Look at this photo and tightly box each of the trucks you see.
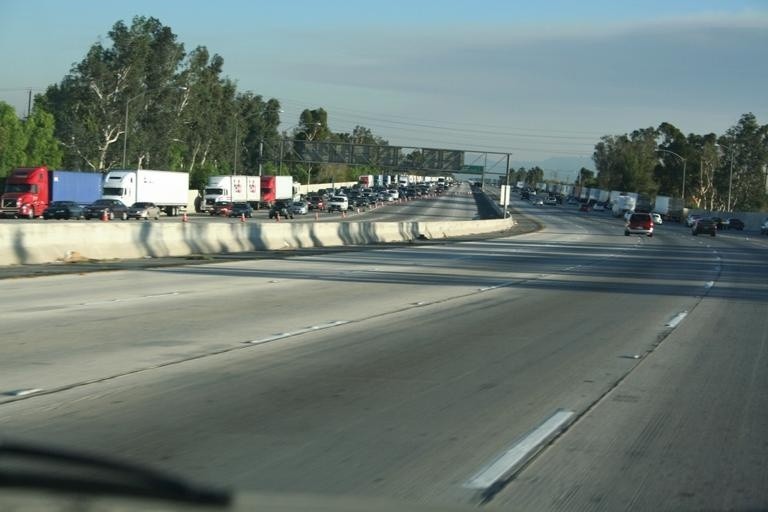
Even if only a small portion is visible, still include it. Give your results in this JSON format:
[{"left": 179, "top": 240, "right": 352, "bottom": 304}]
[
  {"left": 199, "top": 175, "right": 292, "bottom": 213},
  {"left": 359, "top": 175, "right": 453, "bottom": 188},
  {"left": 102, "top": 169, "right": 189, "bottom": 216},
  {"left": 0, "top": 166, "right": 104, "bottom": 218}
]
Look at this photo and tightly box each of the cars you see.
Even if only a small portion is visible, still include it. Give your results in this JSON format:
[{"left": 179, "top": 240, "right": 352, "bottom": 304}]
[
  {"left": 43, "top": 199, "right": 161, "bottom": 220},
  {"left": 269, "top": 182, "right": 464, "bottom": 219},
  {"left": 510, "top": 186, "right": 768, "bottom": 237},
  {"left": 210, "top": 202, "right": 253, "bottom": 218}
]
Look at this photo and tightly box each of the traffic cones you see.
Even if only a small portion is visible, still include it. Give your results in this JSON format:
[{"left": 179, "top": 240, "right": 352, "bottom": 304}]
[
  {"left": 183, "top": 212, "right": 188, "bottom": 222},
  {"left": 276, "top": 213, "right": 281, "bottom": 222},
  {"left": 241, "top": 213, "right": 245, "bottom": 222},
  {"left": 314, "top": 212, "right": 319, "bottom": 220},
  {"left": 101, "top": 211, "right": 108, "bottom": 222}
]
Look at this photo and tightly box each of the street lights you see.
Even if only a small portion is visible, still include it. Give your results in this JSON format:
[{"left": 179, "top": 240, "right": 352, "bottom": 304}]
[
  {"left": 713, "top": 143, "right": 734, "bottom": 212},
  {"left": 654, "top": 148, "right": 686, "bottom": 199},
  {"left": 279, "top": 122, "right": 322, "bottom": 175},
  {"left": 233, "top": 109, "right": 285, "bottom": 175},
  {"left": 121, "top": 87, "right": 188, "bottom": 169}
]
